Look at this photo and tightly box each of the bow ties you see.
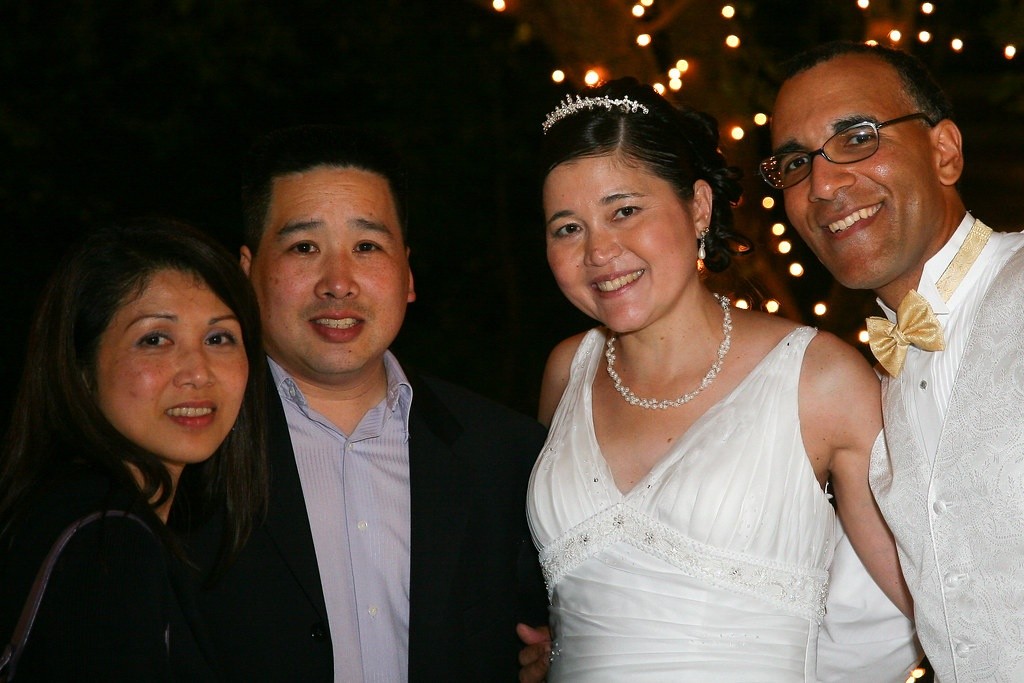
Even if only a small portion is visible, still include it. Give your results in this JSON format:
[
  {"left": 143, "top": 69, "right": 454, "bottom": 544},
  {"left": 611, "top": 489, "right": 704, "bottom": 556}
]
[{"left": 866, "top": 218, "right": 993, "bottom": 378}]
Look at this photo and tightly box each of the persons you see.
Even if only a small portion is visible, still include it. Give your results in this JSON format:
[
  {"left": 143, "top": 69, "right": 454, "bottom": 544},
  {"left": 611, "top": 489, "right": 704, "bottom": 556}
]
[
  {"left": 0, "top": 217, "right": 272, "bottom": 683},
  {"left": 166, "top": 124, "right": 550, "bottom": 683},
  {"left": 523, "top": 76, "right": 914, "bottom": 682},
  {"left": 516, "top": 44, "right": 1024, "bottom": 682}
]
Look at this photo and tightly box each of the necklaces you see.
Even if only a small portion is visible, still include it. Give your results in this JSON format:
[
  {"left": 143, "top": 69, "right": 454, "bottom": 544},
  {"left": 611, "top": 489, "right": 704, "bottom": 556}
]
[{"left": 606, "top": 294, "right": 730, "bottom": 410}]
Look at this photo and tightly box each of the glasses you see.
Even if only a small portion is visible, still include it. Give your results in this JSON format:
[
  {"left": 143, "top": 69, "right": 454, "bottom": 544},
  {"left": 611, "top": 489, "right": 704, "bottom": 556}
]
[{"left": 758, "top": 112, "right": 934, "bottom": 190}]
